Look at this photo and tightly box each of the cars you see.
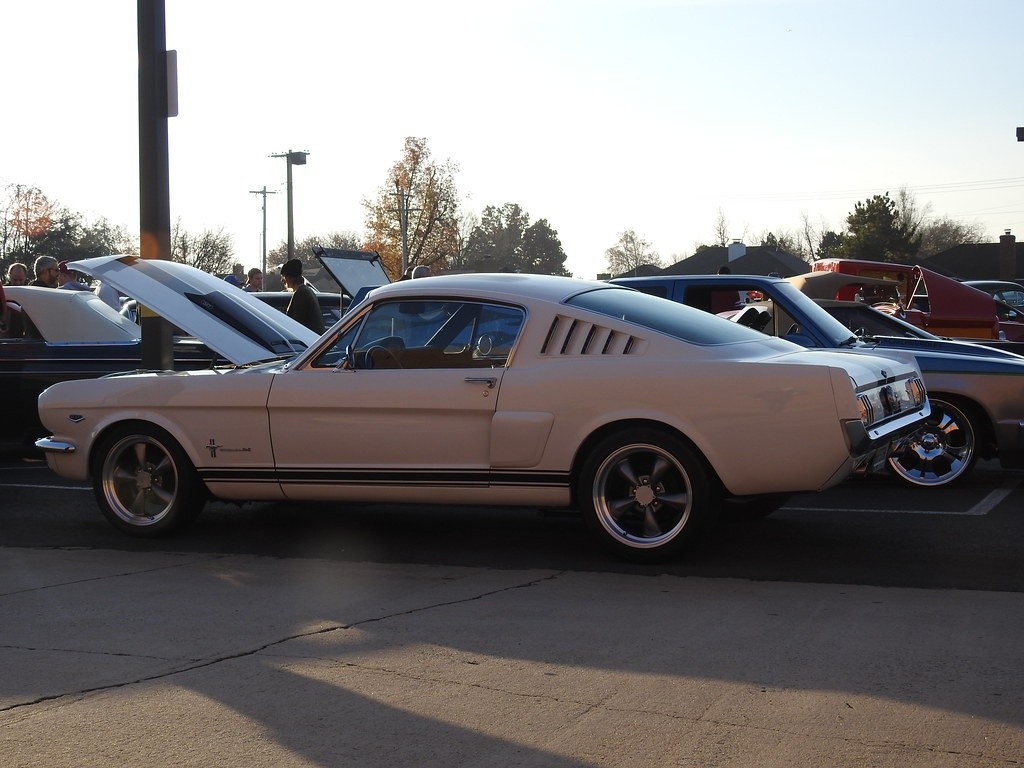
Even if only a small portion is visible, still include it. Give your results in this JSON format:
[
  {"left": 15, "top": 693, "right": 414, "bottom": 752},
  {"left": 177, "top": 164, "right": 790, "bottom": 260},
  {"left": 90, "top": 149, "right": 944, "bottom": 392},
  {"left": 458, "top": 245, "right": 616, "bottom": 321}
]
[
  {"left": 714, "top": 257, "right": 1023, "bottom": 356},
  {"left": 34, "top": 254, "right": 932, "bottom": 540},
  {"left": 0, "top": 286, "right": 231, "bottom": 432},
  {"left": 608, "top": 275, "right": 1023, "bottom": 488}
]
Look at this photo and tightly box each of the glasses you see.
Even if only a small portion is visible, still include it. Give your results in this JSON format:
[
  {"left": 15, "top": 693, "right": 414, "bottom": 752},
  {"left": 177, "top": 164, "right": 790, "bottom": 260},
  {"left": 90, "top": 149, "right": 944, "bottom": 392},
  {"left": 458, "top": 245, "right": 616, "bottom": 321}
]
[{"left": 50, "top": 268, "right": 60, "bottom": 272}]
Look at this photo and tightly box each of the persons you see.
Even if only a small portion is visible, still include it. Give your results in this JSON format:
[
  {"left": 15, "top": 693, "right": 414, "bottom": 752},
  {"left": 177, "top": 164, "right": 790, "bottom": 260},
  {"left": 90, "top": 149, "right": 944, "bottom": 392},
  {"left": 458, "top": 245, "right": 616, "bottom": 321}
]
[
  {"left": 411, "top": 266, "right": 430, "bottom": 279},
  {"left": 20, "top": 256, "right": 59, "bottom": 338},
  {"left": 222, "top": 275, "right": 244, "bottom": 289},
  {"left": 56, "top": 259, "right": 95, "bottom": 292},
  {"left": 241, "top": 268, "right": 262, "bottom": 292},
  {"left": 712, "top": 266, "right": 740, "bottom": 314},
  {"left": 0, "top": 263, "right": 30, "bottom": 337},
  {"left": 395, "top": 266, "right": 414, "bottom": 282},
  {"left": 278, "top": 259, "right": 323, "bottom": 333}
]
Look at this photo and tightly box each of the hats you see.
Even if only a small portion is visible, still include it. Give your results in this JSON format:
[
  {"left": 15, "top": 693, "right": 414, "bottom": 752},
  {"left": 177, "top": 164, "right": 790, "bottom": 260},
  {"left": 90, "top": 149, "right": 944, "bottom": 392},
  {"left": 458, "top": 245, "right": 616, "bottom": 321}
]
[{"left": 280, "top": 258, "right": 303, "bottom": 275}]
